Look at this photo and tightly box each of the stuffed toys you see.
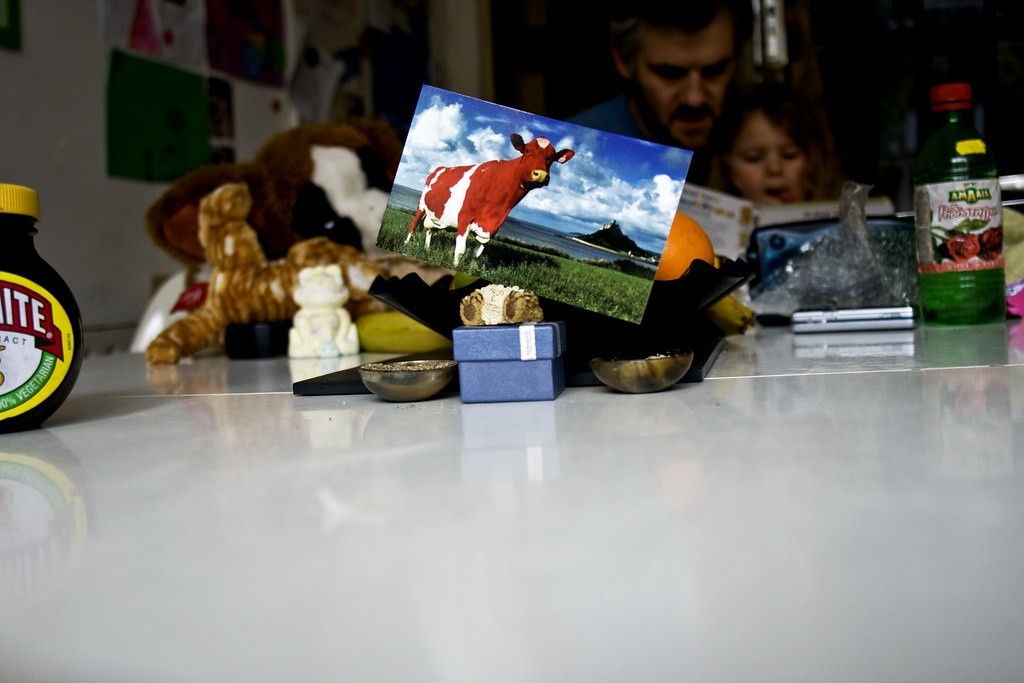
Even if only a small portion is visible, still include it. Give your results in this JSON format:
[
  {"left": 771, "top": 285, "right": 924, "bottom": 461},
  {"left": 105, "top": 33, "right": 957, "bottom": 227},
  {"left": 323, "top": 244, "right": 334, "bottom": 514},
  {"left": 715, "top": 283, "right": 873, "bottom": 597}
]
[{"left": 144, "top": 118, "right": 404, "bottom": 369}]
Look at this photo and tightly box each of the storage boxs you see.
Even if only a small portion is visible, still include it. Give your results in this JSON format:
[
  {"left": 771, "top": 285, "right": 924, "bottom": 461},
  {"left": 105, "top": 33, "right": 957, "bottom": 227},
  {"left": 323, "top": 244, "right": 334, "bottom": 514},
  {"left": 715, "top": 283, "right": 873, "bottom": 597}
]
[{"left": 453, "top": 320, "right": 563, "bottom": 404}]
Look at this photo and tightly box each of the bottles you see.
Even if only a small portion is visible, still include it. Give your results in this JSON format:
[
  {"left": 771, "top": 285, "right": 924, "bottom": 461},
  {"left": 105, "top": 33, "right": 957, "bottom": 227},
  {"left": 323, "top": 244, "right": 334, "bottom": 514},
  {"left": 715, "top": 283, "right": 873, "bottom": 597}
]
[
  {"left": 912, "top": 82, "right": 1006, "bottom": 325},
  {"left": 0, "top": 183, "right": 84, "bottom": 434}
]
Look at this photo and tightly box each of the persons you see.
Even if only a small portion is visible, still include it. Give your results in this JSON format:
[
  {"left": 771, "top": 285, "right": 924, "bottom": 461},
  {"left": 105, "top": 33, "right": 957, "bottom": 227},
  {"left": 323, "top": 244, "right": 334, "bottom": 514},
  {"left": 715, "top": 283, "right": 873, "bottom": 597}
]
[{"left": 567, "top": 1, "right": 844, "bottom": 207}]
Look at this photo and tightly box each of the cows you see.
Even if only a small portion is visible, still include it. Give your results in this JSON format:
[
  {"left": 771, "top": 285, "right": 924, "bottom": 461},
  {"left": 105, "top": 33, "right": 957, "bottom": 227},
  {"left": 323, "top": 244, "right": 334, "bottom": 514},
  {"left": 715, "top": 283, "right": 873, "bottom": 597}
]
[{"left": 403, "top": 133, "right": 575, "bottom": 267}]
